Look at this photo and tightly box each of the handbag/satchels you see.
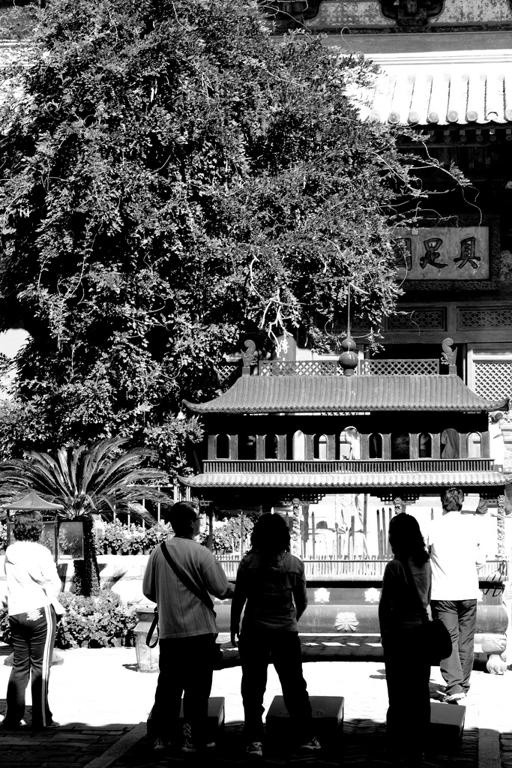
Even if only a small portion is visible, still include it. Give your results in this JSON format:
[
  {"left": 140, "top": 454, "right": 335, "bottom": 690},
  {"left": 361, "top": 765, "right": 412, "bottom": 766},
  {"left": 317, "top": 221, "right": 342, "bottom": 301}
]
[{"left": 420, "top": 617, "right": 452, "bottom": 665}]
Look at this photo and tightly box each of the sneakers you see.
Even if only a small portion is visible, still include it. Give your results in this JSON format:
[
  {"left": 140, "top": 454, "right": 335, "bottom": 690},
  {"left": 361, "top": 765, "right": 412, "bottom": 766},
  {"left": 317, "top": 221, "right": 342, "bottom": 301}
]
[
  {"left": 246, "top": 742, "right": 263, "bottom": 756},
  {"left": 442, "top": 684, "right": 465, "bottom": 702},
  {"left": 303, "top": 736, "right": 322, "bottom": 749}
]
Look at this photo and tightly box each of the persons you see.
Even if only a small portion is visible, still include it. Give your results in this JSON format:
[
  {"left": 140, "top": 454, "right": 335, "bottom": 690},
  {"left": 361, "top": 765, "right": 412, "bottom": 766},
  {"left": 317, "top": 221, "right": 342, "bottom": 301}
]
[
  {"left": 424, "top": 487, "right": 485, "bottom": 704},
  {"left": 0, "top": 511, "right": 62, "bottom": 731},
  {"left": 141, "top": 503, "right": 228, "bottom": 750},
  {"left": 375, "top": 513, "right": 433, "bottom": 756},
  {"left": 230, "top": 512, "right": 324, "bottom": 756}
]
